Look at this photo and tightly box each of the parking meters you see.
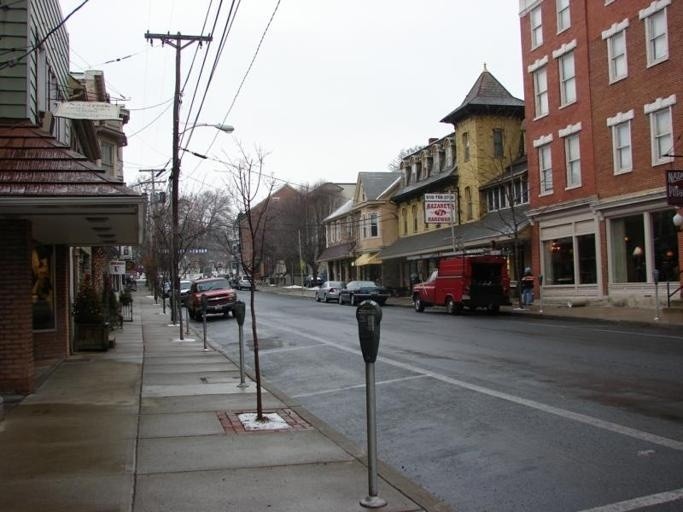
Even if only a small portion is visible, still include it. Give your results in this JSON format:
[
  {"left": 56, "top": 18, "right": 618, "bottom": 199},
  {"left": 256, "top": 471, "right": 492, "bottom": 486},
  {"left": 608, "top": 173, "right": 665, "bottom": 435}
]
[
  {"left": 538, "top": 275, "right": 544, "bottom": 313},
  {"left": 356, "top": 299, "right": 387, "bottom": 508},
  {"left": 232, "top": 300, "right": 246, "bottom": 386},
  {"left": 652, "top": 269, "right": 659, "bottom": 317},
  {"left": 201, "top": 294, "right": 208, "bottom": 349}
]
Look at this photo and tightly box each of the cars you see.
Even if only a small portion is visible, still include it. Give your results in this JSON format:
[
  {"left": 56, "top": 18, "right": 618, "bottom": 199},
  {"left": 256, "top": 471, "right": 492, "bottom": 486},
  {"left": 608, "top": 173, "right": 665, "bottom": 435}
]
[
  {"left": 160, "top": 277, "right": 237, "bottom": 320},
  {"left": 230, "top": 275, "right": 257, "bottom": 291},
  {"left": 303, "top": 275, "right": 325, "bottom": 288},
  {"left": 315, "top": 280, "right": 391, "bottom": 306}
]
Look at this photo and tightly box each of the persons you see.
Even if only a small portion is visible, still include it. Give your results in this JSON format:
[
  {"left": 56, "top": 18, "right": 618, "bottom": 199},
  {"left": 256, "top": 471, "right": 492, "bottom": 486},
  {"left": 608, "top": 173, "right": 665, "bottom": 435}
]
[{"left": 521, "top": 267, "right": 534, "bottom": 305}]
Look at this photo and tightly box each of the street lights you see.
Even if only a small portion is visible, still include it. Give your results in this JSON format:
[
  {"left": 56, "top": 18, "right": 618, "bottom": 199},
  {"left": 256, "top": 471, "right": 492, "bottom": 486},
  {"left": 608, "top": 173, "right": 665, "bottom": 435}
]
[{"left": 178, "top": 124, "right": 234, "bottom": 139}]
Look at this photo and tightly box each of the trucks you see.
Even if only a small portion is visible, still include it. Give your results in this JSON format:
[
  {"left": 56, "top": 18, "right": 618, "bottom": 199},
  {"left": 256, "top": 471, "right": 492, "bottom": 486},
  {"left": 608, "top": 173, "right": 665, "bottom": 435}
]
[{"left": 411, "top": 255, "right": 512, "bottom": 315}]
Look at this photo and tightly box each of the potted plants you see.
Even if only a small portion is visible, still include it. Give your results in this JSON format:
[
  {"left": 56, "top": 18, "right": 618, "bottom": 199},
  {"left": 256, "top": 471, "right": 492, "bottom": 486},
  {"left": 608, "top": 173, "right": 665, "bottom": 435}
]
[{"left": 72, "top": 287, "right": 109, "bottom": 352}]
